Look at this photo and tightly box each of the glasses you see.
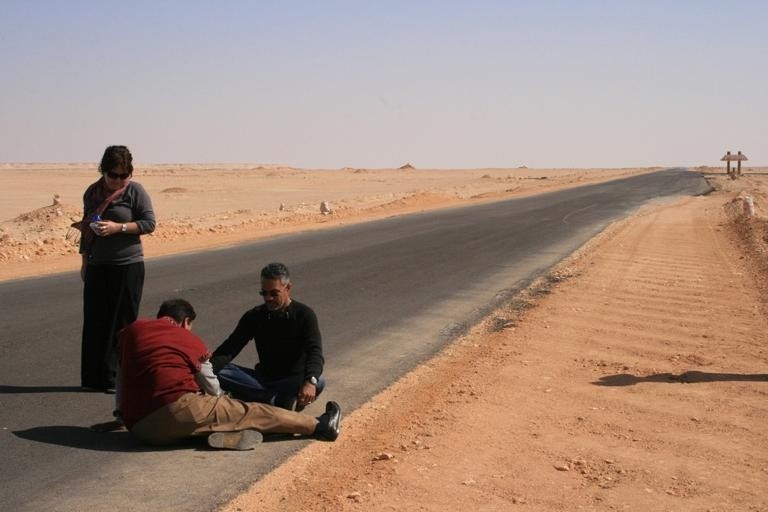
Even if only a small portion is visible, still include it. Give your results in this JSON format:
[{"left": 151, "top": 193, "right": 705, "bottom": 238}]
[
  {"left": 105, "top": 169, "right": 132, "bottom": 181},
  {"left": 258, "top": 283, "right": 289, "bottom": 298}
]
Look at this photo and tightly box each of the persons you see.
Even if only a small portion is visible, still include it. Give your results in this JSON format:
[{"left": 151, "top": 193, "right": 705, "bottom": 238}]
[
  {"left": 209, "top": 261, "right": 326, "bottom": 412},
  {"left": 90, "top": 299, "right": 342, "bottom": 451},
  {"left": 76, "top": 144, "right": 157, "bottom": 394}
]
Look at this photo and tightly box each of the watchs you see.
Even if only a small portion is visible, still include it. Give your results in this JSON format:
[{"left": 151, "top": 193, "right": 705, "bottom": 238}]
[{"left": 310, "top": 376, "right": 318, "bottom": 384}]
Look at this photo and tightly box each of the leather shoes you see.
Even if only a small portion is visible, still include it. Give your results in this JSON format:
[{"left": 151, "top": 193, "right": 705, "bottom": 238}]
[
  {"left": 206, "top": 429, "right": 264, "bottom": 452},
  {"left": 324, "top": 401, "right": 342, "bottom": 442},
  {"left": 275, "top": 392, "right": 298, "bottom": 413},
  {"left": 106, "top": 388, "right": 116, "bottom": 394}
]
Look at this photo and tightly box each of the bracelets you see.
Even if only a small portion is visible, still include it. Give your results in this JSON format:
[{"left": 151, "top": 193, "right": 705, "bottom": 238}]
[{"left": 122, "top": 224, "right": 128, "bottom": 232}]
